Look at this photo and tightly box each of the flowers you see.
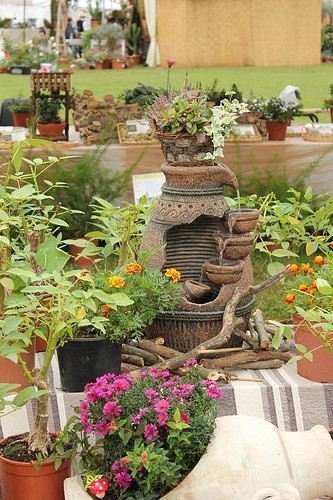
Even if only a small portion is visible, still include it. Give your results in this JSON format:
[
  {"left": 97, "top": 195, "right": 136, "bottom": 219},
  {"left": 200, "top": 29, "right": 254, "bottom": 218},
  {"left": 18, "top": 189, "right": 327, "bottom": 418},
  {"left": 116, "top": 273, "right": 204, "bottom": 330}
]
[
  {"left": 0, "top": 139, "right": 333, "bottom": 500},
  {"left": 248, "top": 94, "right": 300, "bottom": 125},
  {"left": 144, "top": 59, "right": 250, "bottom": 160}
]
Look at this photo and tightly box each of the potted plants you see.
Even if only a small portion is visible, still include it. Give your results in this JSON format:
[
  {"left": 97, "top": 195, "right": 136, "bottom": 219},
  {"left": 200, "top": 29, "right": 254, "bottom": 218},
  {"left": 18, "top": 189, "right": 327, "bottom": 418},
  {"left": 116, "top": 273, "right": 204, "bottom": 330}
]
[
  {"left": 36, "top": 98, "right": 68, "bottom": 136},
  {"left": 321, "top": 32, "right": 333, "bottom": 63},
  {"left": 1, "top": 1, "right": 150, "bottom": 74},
  {"left": 8, "top": 90, "right": 36, "bottom": 128}
]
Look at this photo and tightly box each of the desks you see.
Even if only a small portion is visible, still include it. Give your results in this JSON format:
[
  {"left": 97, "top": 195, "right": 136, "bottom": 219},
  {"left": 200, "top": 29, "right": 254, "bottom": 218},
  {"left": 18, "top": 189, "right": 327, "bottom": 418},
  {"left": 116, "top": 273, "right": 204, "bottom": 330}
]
[
  {"left": 0, "top": 125, "right": 333, "bottom": 206},
  {"left": 0, "top": 324, "right": 333, "bottom": 441}
]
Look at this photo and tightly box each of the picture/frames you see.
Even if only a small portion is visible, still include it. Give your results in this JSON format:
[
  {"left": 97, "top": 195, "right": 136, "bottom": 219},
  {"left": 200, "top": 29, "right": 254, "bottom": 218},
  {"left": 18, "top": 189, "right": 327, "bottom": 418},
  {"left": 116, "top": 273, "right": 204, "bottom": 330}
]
[{"left": 268, "top": 121, "right": 287, "bottom": 141}]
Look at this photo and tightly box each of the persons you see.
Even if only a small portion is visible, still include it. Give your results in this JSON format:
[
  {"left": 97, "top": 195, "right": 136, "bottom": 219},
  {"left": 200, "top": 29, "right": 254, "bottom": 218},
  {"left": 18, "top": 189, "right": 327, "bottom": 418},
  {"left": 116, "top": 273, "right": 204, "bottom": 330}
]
[
  {"left": 65, "top": 17, "right": 77, "bottom": 59},
  {"left": 39, "top": 30, "right": 46, "bottom": 39},
  {"left": 77, "top": 13, "right": 86, "bottom": 58}
]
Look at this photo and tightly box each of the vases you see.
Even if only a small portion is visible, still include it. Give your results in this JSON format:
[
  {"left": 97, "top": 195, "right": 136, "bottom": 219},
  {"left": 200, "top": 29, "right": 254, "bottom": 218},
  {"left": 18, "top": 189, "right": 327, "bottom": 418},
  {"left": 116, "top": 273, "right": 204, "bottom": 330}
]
[
  {"left": 0, "top": 432, "right": 73, "bottom": 500},
  {"left": 0, "top": 335, "right": 36, "bottom": 394},
  {"left": 22, "top": 293, "right": 58, "bottom": 352},
  {"left": 291, "top": 313, "right": 333, "bottom": 383},
  {"left": 57, "top": 336, "right": 123, "bottom": 393},
  {"left": 68, "top": 237, "right": 99, "bottom": 267},
  {"left": 155, "top": 131, "right": 217, "bottom": 167}
]
